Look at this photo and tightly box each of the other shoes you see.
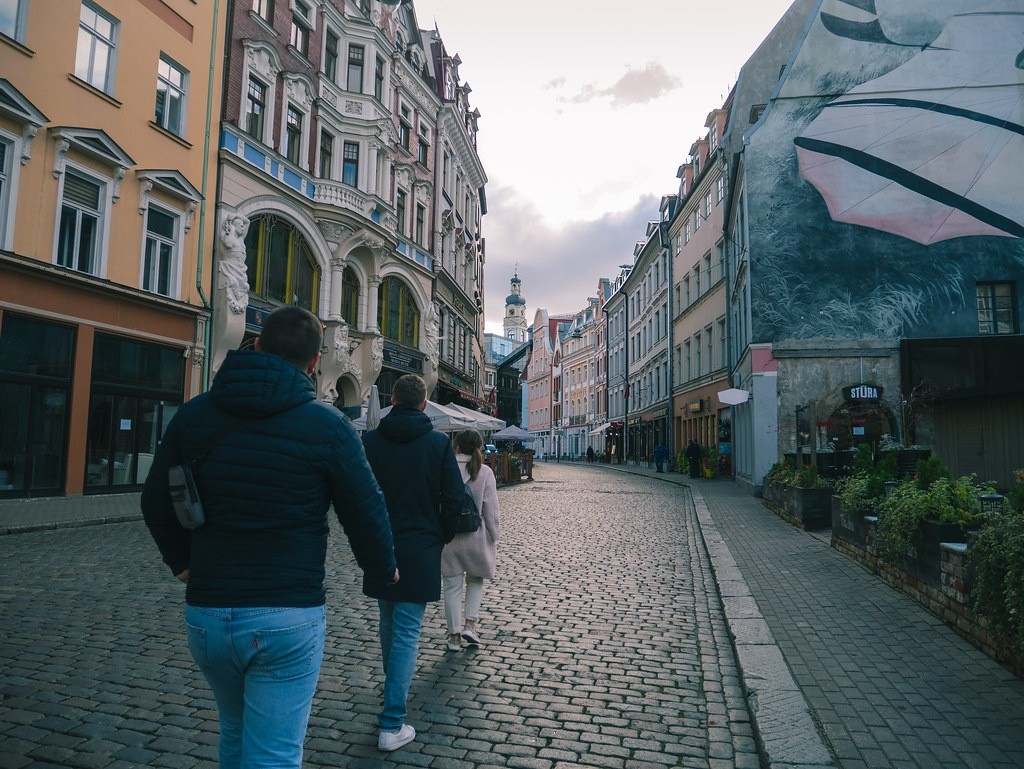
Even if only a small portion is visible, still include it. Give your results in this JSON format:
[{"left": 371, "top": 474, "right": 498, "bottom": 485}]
[
  {"left": 462, "top": 630, "right": 480, "bottom": 644},
  {"left": 446, "top": 639, "right": 460, "bottom": 651}
]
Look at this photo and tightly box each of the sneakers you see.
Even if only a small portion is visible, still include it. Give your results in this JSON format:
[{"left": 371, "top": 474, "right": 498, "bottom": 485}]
[{"left": 378, "top": 722, "right": 416, "bottom": 751}]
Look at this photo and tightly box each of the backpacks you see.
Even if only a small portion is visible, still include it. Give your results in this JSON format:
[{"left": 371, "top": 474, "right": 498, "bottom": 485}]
[{"left": 455, "top": 484, "right": 482, "bottom": 533}]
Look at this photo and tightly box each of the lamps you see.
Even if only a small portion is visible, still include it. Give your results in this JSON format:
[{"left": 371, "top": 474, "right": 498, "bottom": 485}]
[{"left": 347, "top": 341, "right": 361, "bottom": 356}]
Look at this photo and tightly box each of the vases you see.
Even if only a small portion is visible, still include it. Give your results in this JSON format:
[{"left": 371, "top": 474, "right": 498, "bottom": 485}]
[
  {"left": 888, "top": 450, "right": 932, "bottom": 480},
  {"left": 885, "top": 481, "right": 901, "bottom": 498},
  {"left": 921, "top": 516, "right": 981, "bottom": 554}
]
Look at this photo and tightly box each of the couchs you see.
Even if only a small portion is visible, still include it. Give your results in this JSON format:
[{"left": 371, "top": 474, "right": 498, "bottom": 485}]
[{"left": 102, "top": 453, "right": 154, "bottom": 485}]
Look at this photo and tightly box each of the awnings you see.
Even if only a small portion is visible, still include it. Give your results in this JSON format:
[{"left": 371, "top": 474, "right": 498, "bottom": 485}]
[{"left": 589, "top": 422, "right": 612, "bottom": 436}]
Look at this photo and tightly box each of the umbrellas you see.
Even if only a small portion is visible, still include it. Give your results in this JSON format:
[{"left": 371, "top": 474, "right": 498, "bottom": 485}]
[
  {"left": 351, "top": 399, "right": 478, "bottom": 432},
  {"left": 491, "top": 425, "right": 535, "bottom": 455},
  {"left": 444, "top": 402, "right": 506, "bottom": 449}
]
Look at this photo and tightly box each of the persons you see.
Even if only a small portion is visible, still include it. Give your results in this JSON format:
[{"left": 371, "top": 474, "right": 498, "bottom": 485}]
[
  {"left": 692, "top": 439, "right": 702, "bottom": 478},
  {"left": 687, "top": 439, "right": 696, "bottom": 478},
  {"left": 358, "top": 373, "right": 465, "bottom": 752},
  {"left": 653, "top": 442, "right": 665, "bottom": 474},
  {"left": 586, "top": 445, "right": 594, "bottom": 464},
  {"left": 140, "top": 307, "right": 400, "bottom": 769},
  {"left": 441, "top": 429, "right": 500, "bottom": 651}
]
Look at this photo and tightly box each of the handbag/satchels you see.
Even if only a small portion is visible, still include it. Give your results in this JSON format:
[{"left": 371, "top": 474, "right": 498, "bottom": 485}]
[{"left": 167, "top": 463, "right": 205, "bottom": 530}]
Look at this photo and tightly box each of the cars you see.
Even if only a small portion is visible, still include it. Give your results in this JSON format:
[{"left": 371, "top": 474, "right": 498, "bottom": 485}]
[{"left": 485, "top": 445, "right": 499, "bottom": 460}]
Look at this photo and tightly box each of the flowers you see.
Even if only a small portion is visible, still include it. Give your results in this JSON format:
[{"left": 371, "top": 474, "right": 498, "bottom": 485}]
[
  {"left": 872, "top": 472, "right": 1001, "bottom": 557},
  {"left": 878, "top": 433, "right": 934, "bottom": 451}
]
